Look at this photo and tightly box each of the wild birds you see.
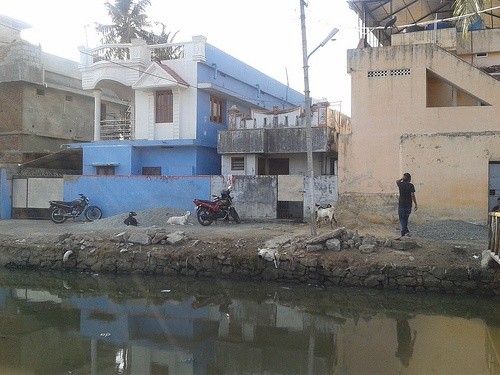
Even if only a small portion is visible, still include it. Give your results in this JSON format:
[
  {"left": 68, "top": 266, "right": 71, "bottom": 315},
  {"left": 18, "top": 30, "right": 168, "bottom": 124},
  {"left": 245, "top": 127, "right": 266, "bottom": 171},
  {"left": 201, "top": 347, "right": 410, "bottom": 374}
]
[{"left": 384, "top": 16, "right": 397, "bottom": 30}]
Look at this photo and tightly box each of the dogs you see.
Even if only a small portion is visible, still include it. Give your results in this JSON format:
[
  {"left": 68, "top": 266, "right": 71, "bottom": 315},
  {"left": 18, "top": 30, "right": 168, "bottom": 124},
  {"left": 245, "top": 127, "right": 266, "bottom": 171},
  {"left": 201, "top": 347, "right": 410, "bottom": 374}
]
[
  {"left": 124, "top": 211, "right": 139, "bottom": 226},
  {"left": 315, "top": 203, "right": 338, "bottom": 229},
  {"left": 167, "top": 211, "right": 193, "bottom": 226}
]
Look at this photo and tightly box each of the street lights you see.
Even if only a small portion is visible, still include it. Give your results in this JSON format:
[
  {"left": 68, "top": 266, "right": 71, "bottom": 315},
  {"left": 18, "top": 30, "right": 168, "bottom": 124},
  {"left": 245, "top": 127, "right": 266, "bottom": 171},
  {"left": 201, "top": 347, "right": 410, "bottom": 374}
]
[{"left": 299, "top": 0, "right": 340, "bottom": 241}]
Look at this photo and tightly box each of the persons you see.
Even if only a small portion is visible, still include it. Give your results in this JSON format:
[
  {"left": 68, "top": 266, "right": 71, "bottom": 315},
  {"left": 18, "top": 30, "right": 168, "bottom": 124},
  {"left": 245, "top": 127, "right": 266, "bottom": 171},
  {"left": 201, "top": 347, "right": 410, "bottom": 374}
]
[
  {"left": 491, "top": 198, "right": 500, "bottom": 211},
  {"left": 396, "top": 172, "right": 418, "bottom": 240}
]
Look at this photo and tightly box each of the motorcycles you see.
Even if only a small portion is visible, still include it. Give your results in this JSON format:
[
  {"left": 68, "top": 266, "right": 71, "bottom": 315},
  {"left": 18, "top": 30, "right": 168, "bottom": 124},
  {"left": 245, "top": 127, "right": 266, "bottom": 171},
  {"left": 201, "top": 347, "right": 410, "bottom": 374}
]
[
  {"left": 193, "top": 185, "right": 242, "bottom": 226},
  {"left": 48, "top": 193, "right": 104, "bottom": 223}
]
